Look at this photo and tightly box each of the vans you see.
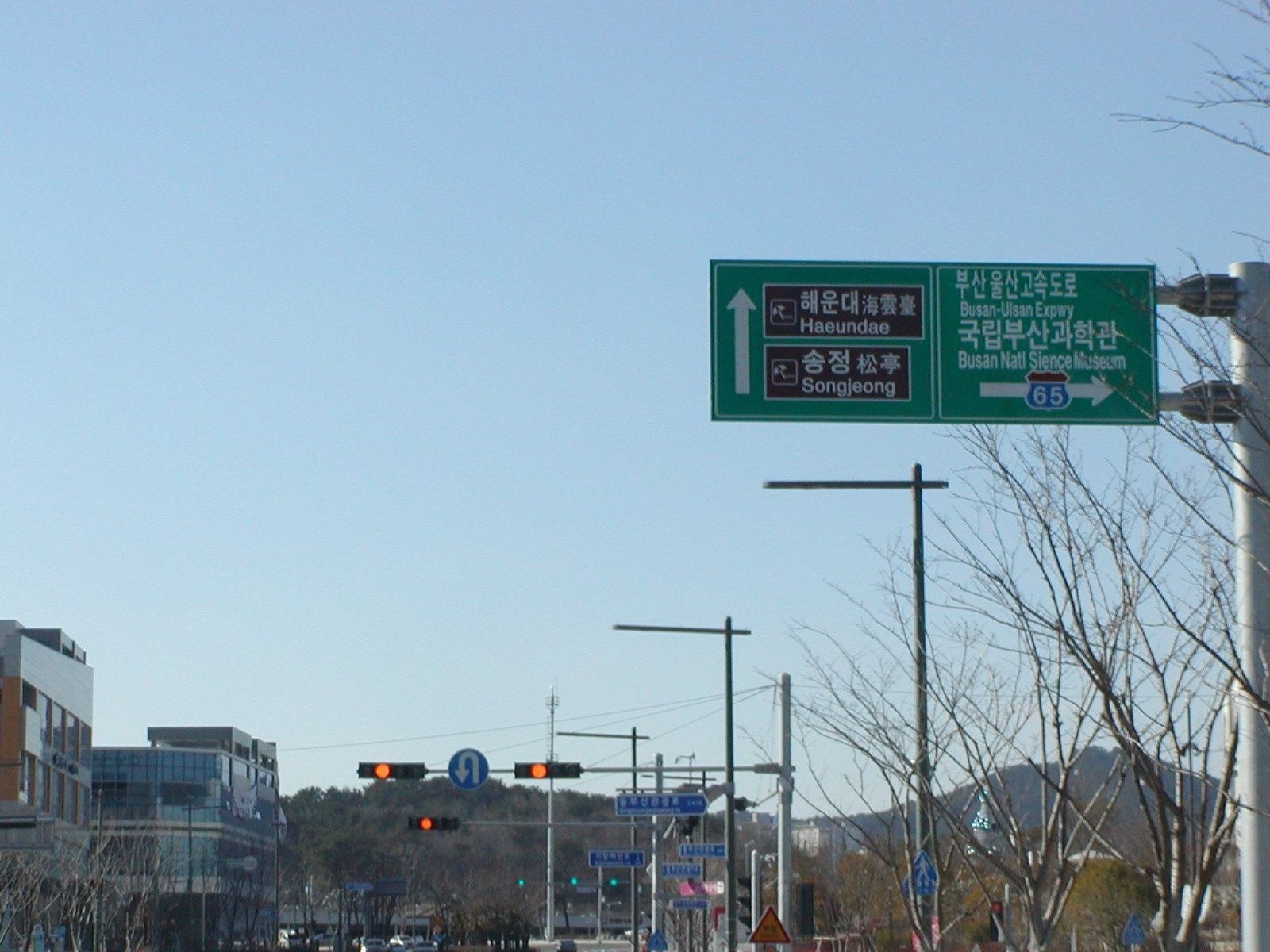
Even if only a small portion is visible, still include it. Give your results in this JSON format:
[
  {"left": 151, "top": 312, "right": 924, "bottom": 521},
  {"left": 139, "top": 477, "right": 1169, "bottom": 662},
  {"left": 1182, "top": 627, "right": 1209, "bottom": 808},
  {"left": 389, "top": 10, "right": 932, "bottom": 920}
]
[{"left": 277, "top": 927, "right": 310, "bottom": 952}]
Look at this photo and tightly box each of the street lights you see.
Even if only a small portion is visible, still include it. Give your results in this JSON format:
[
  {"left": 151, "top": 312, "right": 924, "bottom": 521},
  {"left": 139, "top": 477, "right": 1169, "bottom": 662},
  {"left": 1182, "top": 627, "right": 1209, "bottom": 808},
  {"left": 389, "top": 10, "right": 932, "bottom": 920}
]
[
  {"left": 611, "top": 616, "right": 754, "bottom": 952},
  {"left": 555, "top": 726, "right": 651, "bottom": 952},
  {"left": 760, "top": 462, "right": 949, "bottom": 952},
  {"left": 269, "top": 818, "right": 325, "bottom": 952},
  {"left": 182, "top": 801, "right": 234, "bottom": 952}
]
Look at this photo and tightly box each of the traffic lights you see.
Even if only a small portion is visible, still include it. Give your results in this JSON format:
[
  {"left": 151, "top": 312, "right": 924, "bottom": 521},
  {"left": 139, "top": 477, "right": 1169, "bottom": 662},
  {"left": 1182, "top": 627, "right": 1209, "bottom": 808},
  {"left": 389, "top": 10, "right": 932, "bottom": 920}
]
[
  {"left": 408, "top": 816, "right": 459, "bottom": 831},
  {"left": 357, "top": 761, "right": 425, "bottom": 780},
  {"left": 514, "top": 762, "right": 581, "bottom": 779}
]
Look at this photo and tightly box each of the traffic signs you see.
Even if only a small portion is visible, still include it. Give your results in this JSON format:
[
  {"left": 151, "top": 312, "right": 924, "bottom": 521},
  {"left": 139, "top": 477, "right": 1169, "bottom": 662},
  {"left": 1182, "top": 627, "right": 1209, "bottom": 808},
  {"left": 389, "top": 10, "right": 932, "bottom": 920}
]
[{"left": 707, "top": 257, "right": 1161, "bottom": 428}]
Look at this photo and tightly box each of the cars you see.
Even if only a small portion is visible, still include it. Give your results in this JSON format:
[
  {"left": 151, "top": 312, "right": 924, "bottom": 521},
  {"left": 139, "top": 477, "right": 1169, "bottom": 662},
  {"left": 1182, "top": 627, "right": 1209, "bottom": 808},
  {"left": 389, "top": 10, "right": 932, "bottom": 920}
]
[{"left": 313, "top": 932, "right": 439, "bottom": 952}]
